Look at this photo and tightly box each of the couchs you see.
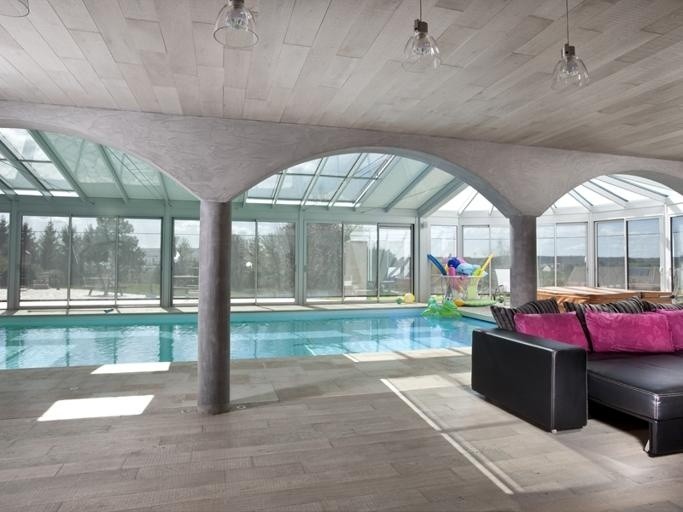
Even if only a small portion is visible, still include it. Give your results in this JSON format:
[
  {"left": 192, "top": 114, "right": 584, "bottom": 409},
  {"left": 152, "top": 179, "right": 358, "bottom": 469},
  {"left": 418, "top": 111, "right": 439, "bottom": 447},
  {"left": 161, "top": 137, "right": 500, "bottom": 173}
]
[{"left": 472, "top": 295, "right": 683, "bottom": 458}]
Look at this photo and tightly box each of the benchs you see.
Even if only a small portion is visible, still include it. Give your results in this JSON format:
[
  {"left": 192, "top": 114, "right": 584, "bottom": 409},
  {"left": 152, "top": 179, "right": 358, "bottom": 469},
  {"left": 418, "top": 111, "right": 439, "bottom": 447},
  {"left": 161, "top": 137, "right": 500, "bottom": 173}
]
[{"left": 81, "top": 277, "right": 126, "bottom": 297}]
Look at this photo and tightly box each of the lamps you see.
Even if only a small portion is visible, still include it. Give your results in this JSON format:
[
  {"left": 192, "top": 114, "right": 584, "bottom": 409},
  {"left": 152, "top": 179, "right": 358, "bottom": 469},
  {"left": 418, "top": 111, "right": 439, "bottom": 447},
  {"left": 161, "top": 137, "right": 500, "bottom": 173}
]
[
  {"left": 550, "top": 1, "right": 591, "bottom": 96},
  {"left": 212, "top": 0, "right": 259, "bottom": 50},
  {"left": 401, "top": 0, "right": 443, "bottom": 74}
]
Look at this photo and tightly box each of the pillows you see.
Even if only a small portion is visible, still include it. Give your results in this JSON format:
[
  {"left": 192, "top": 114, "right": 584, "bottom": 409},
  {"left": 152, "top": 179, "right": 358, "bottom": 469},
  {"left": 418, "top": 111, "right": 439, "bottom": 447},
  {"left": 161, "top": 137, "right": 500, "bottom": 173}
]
[{"left": 489, "top": 294, "right": 683, "bottom": 353}]
